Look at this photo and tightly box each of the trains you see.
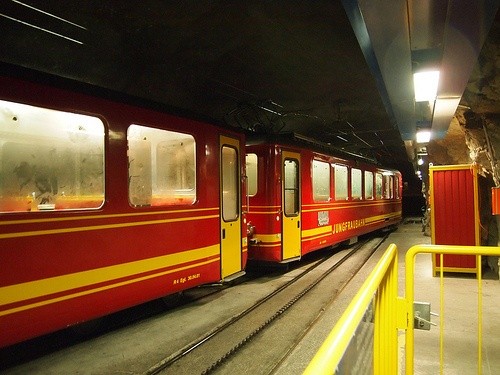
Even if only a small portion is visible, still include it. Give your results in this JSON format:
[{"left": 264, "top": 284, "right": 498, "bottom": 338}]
[{"left": 0, "top": 60, "right": 404, "bottom": 366}]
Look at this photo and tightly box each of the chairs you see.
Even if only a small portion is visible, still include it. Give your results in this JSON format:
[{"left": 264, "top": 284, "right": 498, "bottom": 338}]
[{"left": 0, "top": 194, "right": 195, "bottom": 213}]
